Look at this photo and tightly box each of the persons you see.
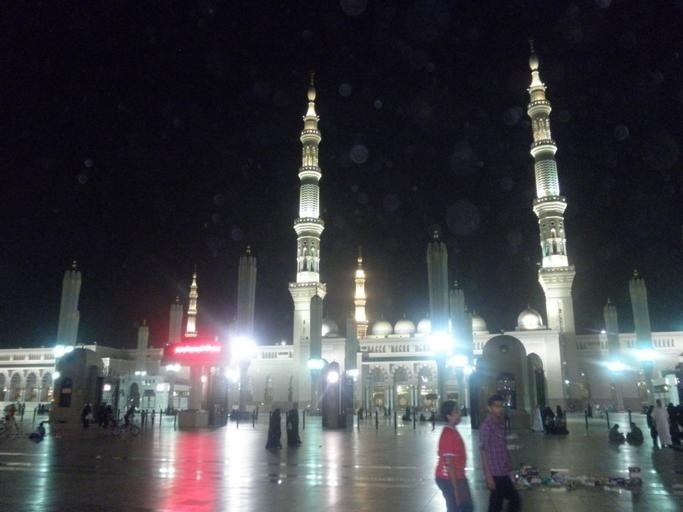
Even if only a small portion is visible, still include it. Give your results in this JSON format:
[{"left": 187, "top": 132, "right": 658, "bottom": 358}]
[
  {"left": 478, "top": 395, "right": 524, "bottom": 512},
  {"left": 542, "top": 406, "right": 555, "bottom": 433},
  {"left": 609, "top": 423, "right": 624, "bottom": 441},
  {"left": 2, "top": 396, "right": 158, "bottom": 443},
  {"left": 652, "top": 399, "right": 673, "bottom": 448},
  {"left": 646, "top": 405, "right": 659, "bottom": 449},
  {"left": 667, "top": 401, "right": 683, "bottom": 445},
  {"left": 531, "top": 403, "right": 542, "bottom": 431},
  {"left": 433, "top": 400, "right": 474, "bottom": 512},
  {"left": 555, "top": 404, "right": 570, "bottom": 435},
  {"left": 266, "top": 410, "right": 282, "bottom": 449},
  {"left": 285, "top": 401, "right": 303, "bottom": 446},
  {"left": 626, "top": 421, "right": 643, "bottom": 443}
]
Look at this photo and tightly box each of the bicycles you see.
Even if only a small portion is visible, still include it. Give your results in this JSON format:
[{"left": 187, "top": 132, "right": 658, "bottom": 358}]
[{"left": 111, "top": 422, "right": 140, "bottom": 436}]
